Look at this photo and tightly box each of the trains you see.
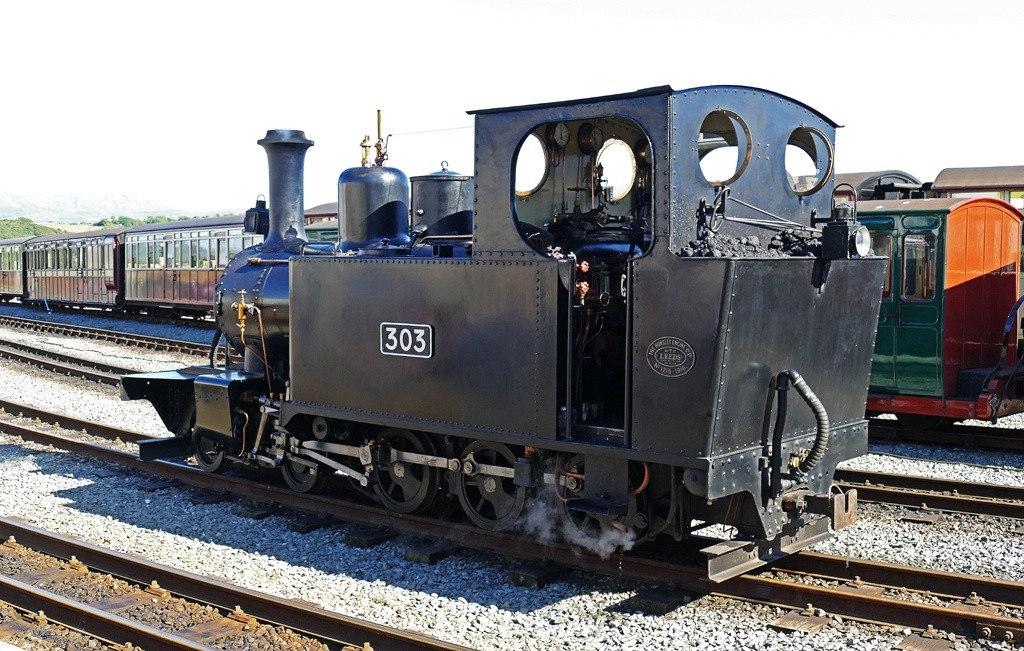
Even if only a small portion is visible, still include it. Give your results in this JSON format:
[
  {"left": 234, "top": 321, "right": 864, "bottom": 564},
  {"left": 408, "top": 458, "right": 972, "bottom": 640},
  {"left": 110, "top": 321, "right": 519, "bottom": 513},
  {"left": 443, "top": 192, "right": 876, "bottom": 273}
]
[
  {"left": 140, "top": 84, "right": 892, "bottom": 585},
  {"left": 0, "top": 162, "right": 1024, "bottom": 448}
]
